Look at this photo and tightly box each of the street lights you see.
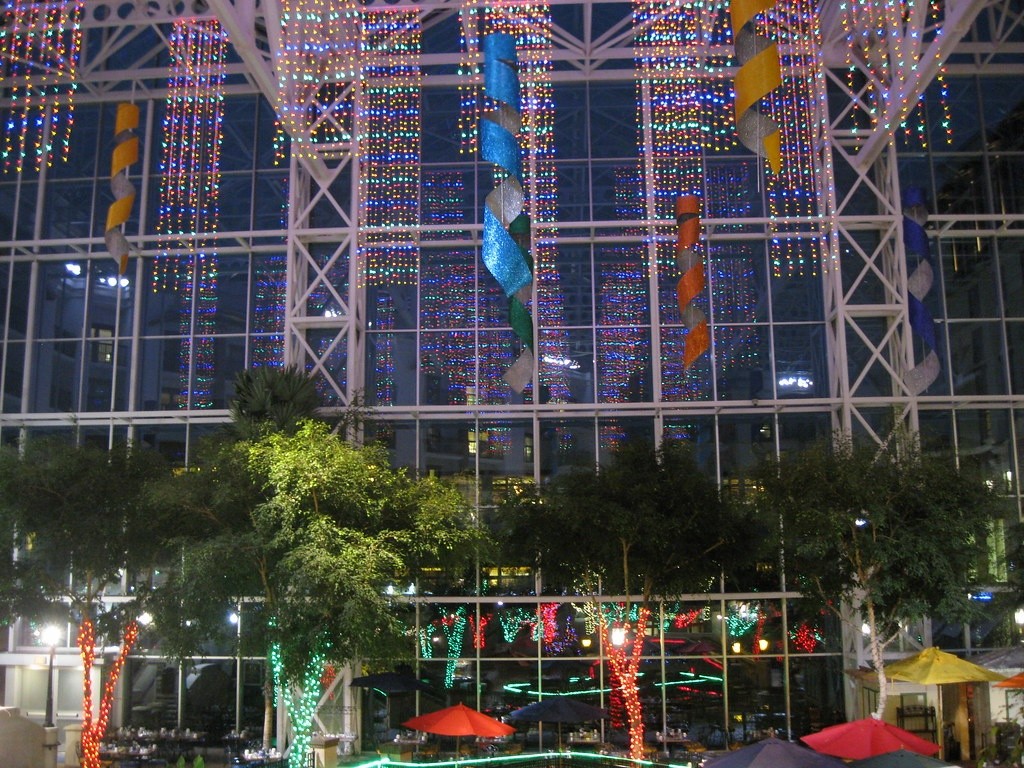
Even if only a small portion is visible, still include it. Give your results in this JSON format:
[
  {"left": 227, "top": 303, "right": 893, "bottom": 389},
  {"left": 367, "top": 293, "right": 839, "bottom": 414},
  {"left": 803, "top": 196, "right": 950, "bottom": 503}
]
[{"left": 40, "top": 625, "right": 62, "bottom": 727}]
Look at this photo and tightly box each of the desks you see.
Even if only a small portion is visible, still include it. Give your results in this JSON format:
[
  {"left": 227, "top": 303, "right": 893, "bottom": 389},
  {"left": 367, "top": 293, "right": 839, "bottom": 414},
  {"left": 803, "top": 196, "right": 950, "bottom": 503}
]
[
  {"left": 221, "top": 734, "right": 248, "bottom": 752},
  {"left": 99, "top": 747, "right": 160, "bottom": 767},
  {"left": 108, "top": 731, "right": 209, "bottom": 761}
]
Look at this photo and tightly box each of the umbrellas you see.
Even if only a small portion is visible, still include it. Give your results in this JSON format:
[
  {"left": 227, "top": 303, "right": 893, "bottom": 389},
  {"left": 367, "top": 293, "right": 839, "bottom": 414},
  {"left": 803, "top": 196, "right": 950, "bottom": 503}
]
[
  {"left": 351, "top": 672, "right": 432, "bottom": 728},
  {"left": 849, "top": 744, "right": 961, "bottom": 768},
  {"left": 509, "top": 694, "right": 609, "bottom": 749},
  {"left": 703, "top": 730, "right": 850, "bottom": 768},
  {"left": 800, "top": 712, "right": 940, "bottom": 760},
  {"left": 402, "top": 701, "right": 517, "bottom": 757},
  {"left": 857, "top": 646, "right": 1009, "bottom": 760},
  {"left": 995, "top": 673, "right": 1024, "bottom": 688}
]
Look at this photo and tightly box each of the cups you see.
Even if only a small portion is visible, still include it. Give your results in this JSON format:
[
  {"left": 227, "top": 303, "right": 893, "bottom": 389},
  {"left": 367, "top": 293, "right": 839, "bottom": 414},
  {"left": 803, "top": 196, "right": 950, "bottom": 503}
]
[
  {"left": 185, "top": 728, "right": 190, "bottom": 736},
  {"left": 569, "top": 729, "right": 600, "bottom": 742},
  {"left": 161, "top": 727, "right": 166, "bottom": 736},
  {"left": 244, "top": 747, "right": 281, "bottom": 759},
  {"left": 393, "top": 732, "right": 428, "bottom": 743},
  {"left": 655, "top": 729, "right": 687, "bottom": 740}
]
